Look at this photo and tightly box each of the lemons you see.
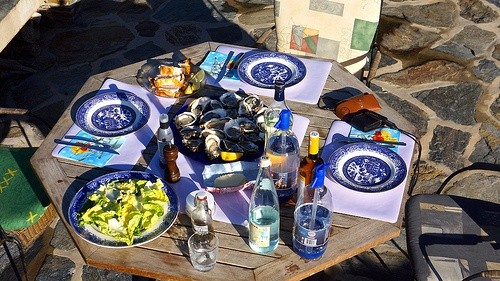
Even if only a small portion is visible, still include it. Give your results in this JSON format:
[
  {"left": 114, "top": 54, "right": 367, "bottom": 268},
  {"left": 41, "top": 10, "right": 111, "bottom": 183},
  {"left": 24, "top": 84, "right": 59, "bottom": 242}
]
[
  {"left": 185, "top": 70, "right": 204, "bottom": 94},
  {"left": 220, "top": 151, "right": 243, "bottom": 161}
]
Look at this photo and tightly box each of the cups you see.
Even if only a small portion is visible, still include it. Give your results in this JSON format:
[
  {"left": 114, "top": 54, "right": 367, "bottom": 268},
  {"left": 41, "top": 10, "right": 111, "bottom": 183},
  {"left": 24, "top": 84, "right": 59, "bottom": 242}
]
[
  {"left": 188, "top": 232, "right": 219, "bottom": 272},
  {"left": 186, "top": 190, "right": 215, "bottom": 221}
]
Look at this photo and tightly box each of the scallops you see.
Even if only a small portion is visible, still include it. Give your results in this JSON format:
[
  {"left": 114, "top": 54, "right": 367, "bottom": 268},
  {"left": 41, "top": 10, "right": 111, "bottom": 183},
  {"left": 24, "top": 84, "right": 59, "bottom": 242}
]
[{"left": 172, "top": 92, "right": 267, "bottom": 161}]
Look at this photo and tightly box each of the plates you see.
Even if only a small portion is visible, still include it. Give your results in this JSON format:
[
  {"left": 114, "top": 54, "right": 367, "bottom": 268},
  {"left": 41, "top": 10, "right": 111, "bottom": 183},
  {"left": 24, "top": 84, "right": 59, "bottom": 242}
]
[
  {"left": 327, "top": 142, "right": 408, "bottom": 193},
  {"left": 136, "top": 59, "right": 205, "bottom": 98},
  {"left": 236, "top": 52, "right": 306, "bottom": 88},
  {"left": 76, "top": 91, "right": 150, "bottom": 137},
  {"left": 61, "top": 164, "right": 180, "bottom": 248},
  {"left": 170, "top": 96, "right": 269, "bottom": 164}
]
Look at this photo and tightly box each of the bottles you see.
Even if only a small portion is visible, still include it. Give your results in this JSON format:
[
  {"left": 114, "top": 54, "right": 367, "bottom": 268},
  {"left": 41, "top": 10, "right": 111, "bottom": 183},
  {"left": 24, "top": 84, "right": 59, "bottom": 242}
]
[
  {"left": 248, "top": 156, "right": 280, "bottom": 254},
  {"left": 155, "top": 114, "right": 175, "bottom": 165},
  {"left": 297, "top": 131, "right": 324, "bottom": 205},
  {"left": 292, "top": 162, "right": 332, "bottom": 258},
  {"left": 266, "top": 110, "right": 300, "bottom": 202},
  {"left": 163, "top": 142, "right": 180, "bottom": 183},
  {"left": 188, "top": 192, "right": 220, "bottom": 242},
  {"left": 264, "top": 81, "right": 293, "bottom": 150}
]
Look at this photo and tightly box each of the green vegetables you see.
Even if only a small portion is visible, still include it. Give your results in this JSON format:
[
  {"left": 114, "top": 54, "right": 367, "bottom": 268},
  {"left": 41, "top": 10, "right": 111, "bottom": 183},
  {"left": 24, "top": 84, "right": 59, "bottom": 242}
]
[{"left": 79, "top": 179, "right": 169, "bottom": 246}]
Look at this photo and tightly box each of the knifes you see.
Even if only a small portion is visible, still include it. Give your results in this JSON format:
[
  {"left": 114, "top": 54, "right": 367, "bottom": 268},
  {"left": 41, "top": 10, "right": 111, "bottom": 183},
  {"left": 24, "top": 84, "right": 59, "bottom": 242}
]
[
  {"left": 54, "top": 138, "right": 120, "bottom": 155},
  {"left": 337, "top": 138, "right": 406, "bottom": 146},
  {"left": 214, "top": 51, "right": 234, "bottom": 84}
]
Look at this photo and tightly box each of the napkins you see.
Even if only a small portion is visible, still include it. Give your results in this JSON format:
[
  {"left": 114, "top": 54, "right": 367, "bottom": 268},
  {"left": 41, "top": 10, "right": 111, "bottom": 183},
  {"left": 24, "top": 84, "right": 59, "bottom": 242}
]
[
  {"left": 59, "top": 130, "right": 126, "bottom": 168},
  {"left": 200, "top": 51, "right": 241, "bottom": 80},
  {"left": 349, "top": 126, "right": 400, "bottom": 154}
]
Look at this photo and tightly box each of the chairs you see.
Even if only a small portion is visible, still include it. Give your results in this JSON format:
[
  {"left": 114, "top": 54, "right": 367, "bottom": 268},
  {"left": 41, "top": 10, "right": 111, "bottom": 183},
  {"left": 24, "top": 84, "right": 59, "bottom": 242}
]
[
  {"left": 255, "top": 0, "right": 383, "bottom": 87},
  {"left": 0, "top": 107, "right": 57, "bottom": 281},
  {"left": 404, "top": 165, "right": 500, "bottom": 281}
]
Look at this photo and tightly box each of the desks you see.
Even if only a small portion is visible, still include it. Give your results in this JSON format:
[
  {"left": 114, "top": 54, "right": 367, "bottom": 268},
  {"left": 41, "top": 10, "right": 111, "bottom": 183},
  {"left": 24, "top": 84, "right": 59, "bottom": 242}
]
[{"left": 29, "top": 42, "right": 418, "bottom": 281}]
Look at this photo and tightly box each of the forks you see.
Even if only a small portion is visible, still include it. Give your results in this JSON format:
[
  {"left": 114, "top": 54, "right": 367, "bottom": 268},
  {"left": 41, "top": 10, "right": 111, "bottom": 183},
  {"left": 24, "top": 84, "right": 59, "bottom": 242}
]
[
  {"left": 65, "top": 135, "right": 123, "bottom": 149},
  {"left": 222, "top": 52, "right": 244, "bottom": 78}
]
